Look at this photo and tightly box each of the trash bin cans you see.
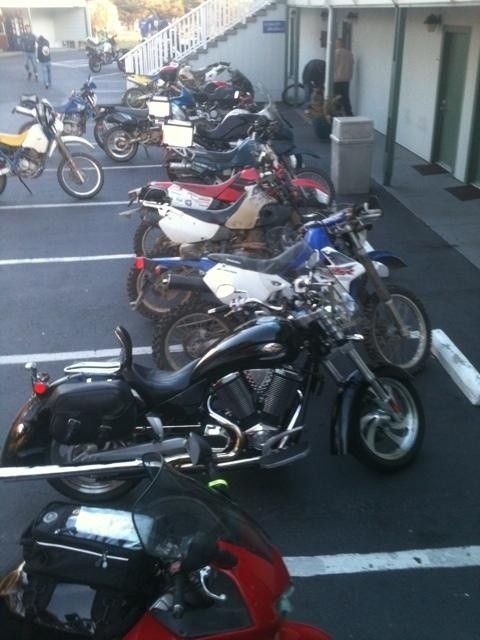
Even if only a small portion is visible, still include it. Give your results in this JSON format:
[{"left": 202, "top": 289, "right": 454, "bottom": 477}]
[{"left": 329, "top": 116, "right": 375, "bottom": 195}]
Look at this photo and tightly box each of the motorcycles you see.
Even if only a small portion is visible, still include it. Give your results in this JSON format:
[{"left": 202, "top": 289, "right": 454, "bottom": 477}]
[
  {"left": 0, "top": 93, "right": 103, "bottom": 199},
  {"left": 0, "top": 250, "right": 426, "bottom": 505},
  {"left": 0, "top": 431, "right": 331, "bottom": 640},
  {"left": 17, "top": 72, "right": 123, "bottom": 148}
]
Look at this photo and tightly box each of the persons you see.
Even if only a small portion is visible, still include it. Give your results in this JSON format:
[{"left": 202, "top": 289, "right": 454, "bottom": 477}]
[
  {"left": 21, "top": 24, "right": 41, "bottom": 81},
  {"left": 36, "top": 35, "right": 52, "bottom": 88},
  {"left": 138, "top": 8, "right": 169, "bottom": 43},
  {"left": 332, "top": 37, "right": 354, "bottom": 117},
  {"left": 302, "top": 58, "right": 326, "bottom": 114}
]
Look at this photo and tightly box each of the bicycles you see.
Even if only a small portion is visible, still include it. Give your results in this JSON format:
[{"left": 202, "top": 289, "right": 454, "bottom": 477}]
[{"left": 89, "top": 33, "right": 129, "bottom": 72}]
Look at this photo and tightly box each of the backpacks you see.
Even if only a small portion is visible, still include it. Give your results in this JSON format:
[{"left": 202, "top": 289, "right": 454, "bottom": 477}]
[{"left": 18, "top": 501, "right": 155, "bottom": 596}]
